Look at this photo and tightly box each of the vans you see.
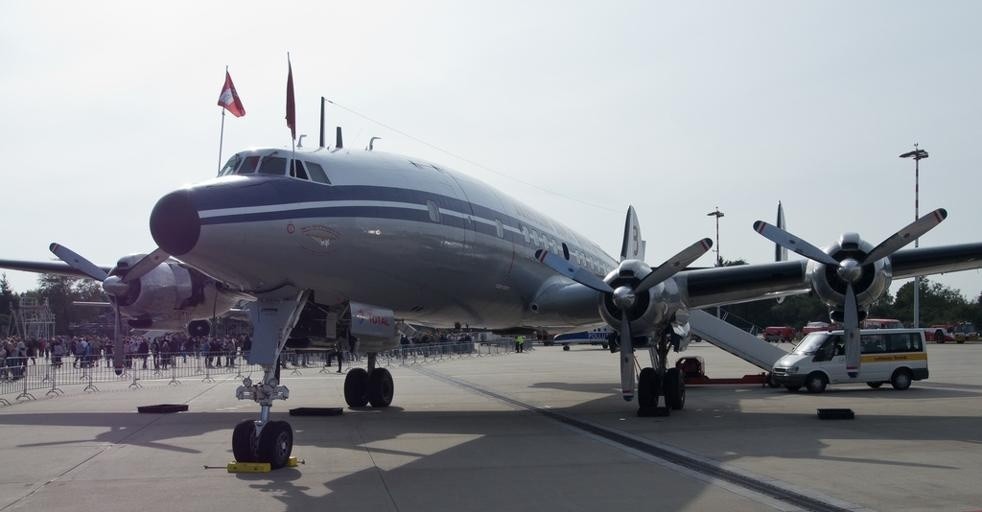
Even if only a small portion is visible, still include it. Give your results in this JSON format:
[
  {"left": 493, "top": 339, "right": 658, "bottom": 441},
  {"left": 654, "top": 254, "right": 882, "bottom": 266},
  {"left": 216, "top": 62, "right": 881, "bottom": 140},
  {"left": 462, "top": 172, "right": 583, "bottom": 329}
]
[{"left": 771, "top": 328, "right": 929, "bottom": 393}]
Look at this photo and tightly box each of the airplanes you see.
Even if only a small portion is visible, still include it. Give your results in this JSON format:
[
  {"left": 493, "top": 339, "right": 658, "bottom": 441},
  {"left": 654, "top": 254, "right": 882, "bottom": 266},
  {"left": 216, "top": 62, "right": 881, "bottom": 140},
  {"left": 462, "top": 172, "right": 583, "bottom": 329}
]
[
  {"left": 0, "top": 98, "right": 978, "bottom": 468},
  {"left": 551, "top": 322, "right": 616, "bottom": 351}
]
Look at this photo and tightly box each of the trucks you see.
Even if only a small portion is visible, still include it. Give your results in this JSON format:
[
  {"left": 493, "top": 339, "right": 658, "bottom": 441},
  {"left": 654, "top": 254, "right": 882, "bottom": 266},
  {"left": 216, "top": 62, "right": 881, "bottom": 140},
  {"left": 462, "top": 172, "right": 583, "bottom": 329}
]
[
  {"left": 762, "top": 326, "right": 796, "bottom": 343},
  {"left": 864, "top": 317, "right": 904, "bottom": 344},
  {"left": 802, "top": 320, "right": 841, "bottom": 335},
  {"left": 923, "top": 321, "right": 976, "bottom": 343}
]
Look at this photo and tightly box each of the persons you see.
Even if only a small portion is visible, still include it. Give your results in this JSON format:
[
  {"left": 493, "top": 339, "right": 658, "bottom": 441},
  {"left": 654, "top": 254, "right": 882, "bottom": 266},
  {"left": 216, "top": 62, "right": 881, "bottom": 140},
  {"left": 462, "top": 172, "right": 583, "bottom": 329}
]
[
  {"left": 400, "top": 328, "right": 472, "bottom": 358},
  {"left": 515, "top": 336, "right": 526, "bottom": 352},
  {"left": 324, "top": 338, "right": 344, "bottom": 373},
  {"left": 0, "top": 332, "right": 253, "bottom": 382}
]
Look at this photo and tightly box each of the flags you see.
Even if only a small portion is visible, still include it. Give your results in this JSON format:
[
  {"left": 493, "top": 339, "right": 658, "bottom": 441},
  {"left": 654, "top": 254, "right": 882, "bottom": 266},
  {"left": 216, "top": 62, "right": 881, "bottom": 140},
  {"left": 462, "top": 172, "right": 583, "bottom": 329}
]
[
  {"left": 285, "top": 60, "right": 295, "bottom": 137},
  {"left": 217, "top": 71, "right": 245, "bottom": 118}
]
